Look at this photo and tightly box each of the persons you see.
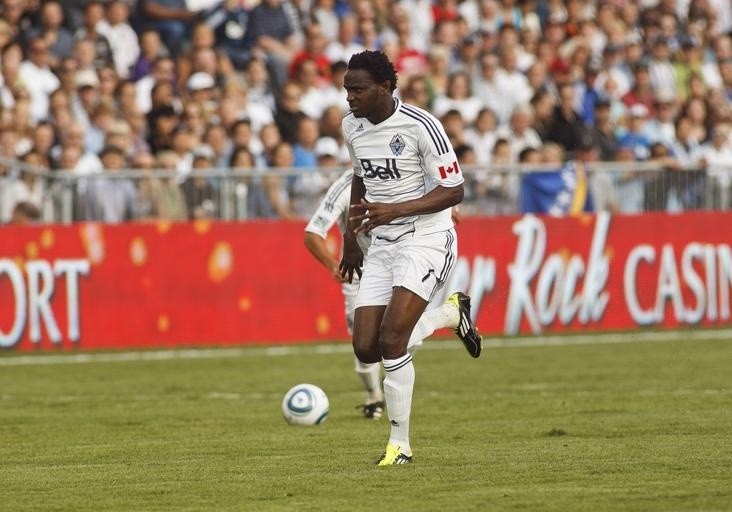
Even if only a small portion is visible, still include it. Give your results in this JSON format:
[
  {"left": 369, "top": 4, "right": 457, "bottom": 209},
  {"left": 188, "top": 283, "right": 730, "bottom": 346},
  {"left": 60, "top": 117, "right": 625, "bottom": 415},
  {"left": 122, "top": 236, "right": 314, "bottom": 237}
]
[
  {"left": 0, "top": 0, "right": 732, "bottom": 227},
  {"left": 304, "top": 167, "right": 424, "bottom": 421},
  {"left": 337, "top": 51, "right": 483, "bottom": 468}
]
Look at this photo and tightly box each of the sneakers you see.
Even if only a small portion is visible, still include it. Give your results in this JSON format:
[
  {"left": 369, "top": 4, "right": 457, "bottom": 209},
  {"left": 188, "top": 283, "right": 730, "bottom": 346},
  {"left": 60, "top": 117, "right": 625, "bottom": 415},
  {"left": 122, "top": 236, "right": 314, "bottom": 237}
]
[
  {"left": 374, "top": 445, "right": 413, "bottom": 466},
  {"left": 445, "top": 292, "right": 482, "bottom": 359},
  {"left": 364, "top": 400, "right": 384, "bottom": 420}
]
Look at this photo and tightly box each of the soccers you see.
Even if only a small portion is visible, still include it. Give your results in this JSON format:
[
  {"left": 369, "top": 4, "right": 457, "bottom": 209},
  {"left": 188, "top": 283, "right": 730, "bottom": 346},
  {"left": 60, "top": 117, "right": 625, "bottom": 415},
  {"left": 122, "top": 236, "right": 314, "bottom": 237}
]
[{"left": 282, "top": 383, "right": 330, "bottom": 425}]
